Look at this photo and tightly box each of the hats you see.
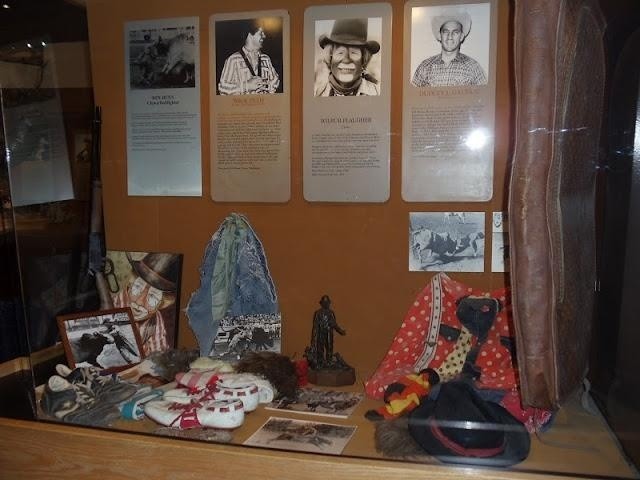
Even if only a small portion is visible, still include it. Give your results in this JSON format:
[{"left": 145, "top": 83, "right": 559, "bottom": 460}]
[
  {"left": 430, "top": 6, "right": 472, "bottom": 42},
  {"left": 317, "top": 18, "right": 381, "bottom": 56},
  {"left": 123, "top": 251, "right": 183, "bottom": 295},
  {"left": 407, "top": 381, "right": 533, "bottom": 471}
]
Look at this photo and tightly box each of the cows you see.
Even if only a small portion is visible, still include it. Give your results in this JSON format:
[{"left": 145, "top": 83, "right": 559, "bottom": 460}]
[
  {"left": 67, "top": 330, "right": 116, "bottom": 371},
  {"left": 409, "top": 228, "right": 465, "bottom": 264},
  {"left": 441, "top": 212, "right": 467, "bottom": 228}
]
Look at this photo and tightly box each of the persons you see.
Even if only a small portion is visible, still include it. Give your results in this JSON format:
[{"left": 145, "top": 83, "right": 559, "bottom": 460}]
[
  {"left": 312, "top": 15, "right": 383, "bottom": 100},
  {"left": 451, "top": 229, "right": 484, "bottom": 260},
  {"left": 108, "top": 254, "right": 183, "bottom": 356},
  {"left": 217, "top": 20, "right": 281, "bottom": 98},
  {"left": 309, "top": 294, "right": 347, "bottom": 369},
  {"left": 101, "top": 317, "right": 140, "bottom": 365},
  {"left": 412, "top": 18, "right": 488, "bottom": 88}
]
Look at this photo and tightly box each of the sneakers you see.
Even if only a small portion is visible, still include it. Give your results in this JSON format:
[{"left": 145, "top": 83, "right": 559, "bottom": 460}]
[
  {"left": 40, "top": 361, "right": 137, "bottom": 431},
  {"left": 144, "top": 356, "right": 277, "bottom": 433}
]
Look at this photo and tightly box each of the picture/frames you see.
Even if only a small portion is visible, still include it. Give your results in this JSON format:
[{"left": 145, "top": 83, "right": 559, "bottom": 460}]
[{"left": 55, "top": 306, "right": 146, "bottom": 377}]
